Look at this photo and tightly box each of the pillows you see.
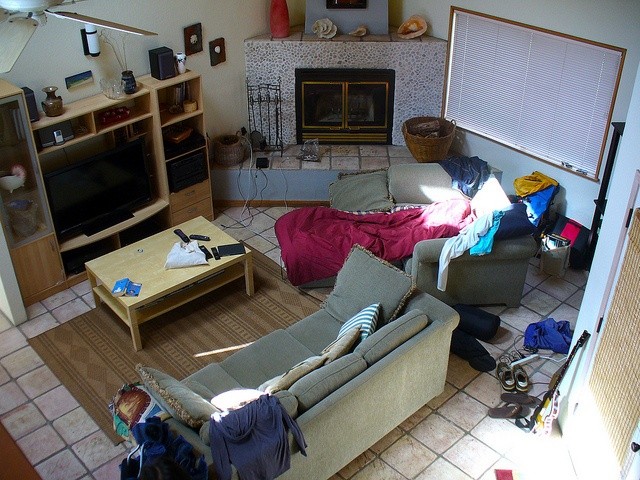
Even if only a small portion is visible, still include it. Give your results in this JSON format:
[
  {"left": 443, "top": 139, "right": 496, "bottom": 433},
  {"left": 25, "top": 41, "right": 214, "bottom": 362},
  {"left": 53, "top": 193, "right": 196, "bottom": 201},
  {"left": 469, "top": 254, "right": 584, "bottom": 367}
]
[
  {"left": 337, "top": 304, "right": 382, "bottom": 341},
  {"left": 212, "top": 382, "right": 260, "bottom": 411},
  {"left": 330, "top": 168, "right": 394, "bottom": 216},
  {"left": 258, "top": 347, "right": 327, "bottom": 395},
  {"left": 320, "top": 324, "right": 368, "bottom": 365},
  {"left": 320, "top": 242, "right": 415, "bottom": 329},
  {"left": 132, "top": 362, "right": 220, "bottom": 426}
]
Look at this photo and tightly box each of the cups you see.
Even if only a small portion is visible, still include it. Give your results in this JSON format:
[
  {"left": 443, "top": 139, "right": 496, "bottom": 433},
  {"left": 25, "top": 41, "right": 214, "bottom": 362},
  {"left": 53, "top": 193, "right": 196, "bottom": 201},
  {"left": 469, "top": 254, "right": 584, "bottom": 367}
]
[{"left": 100, "top": 79, "right": 125, "bottom": 97}]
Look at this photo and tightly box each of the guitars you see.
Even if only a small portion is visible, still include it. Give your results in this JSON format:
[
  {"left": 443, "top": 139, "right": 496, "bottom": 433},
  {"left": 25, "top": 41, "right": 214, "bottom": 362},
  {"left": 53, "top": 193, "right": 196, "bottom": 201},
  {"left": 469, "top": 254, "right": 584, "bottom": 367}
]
[{"left": 527, "top": 330, "right": 592, "bottom": 437}]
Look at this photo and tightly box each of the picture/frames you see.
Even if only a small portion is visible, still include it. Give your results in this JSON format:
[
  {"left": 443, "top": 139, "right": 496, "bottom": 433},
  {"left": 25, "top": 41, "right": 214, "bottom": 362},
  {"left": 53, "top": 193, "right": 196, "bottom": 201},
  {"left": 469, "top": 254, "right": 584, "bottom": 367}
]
[
  {"left": 209, "top": 38, "right": 226, "bottom": 66},
  {"left": 184, "top": 22, "right": 203, "bottom": 56}
]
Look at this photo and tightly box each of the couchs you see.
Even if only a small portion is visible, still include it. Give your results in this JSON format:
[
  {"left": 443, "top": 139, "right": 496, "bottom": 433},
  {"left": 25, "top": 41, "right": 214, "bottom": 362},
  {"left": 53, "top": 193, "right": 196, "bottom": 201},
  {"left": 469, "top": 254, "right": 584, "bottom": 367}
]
[
  {"left": 391, "top": 158, "right": 537, "bottom": 309},
  {"left": 109, "top": 244, "right": 461, "bottom": 479}
]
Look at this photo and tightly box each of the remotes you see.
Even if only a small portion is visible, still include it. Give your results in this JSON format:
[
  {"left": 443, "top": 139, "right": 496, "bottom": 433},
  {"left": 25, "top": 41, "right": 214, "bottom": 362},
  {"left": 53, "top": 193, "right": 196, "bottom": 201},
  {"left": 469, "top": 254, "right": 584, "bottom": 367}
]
[
  {"left": 189, "top": 234, "right": 210, "bottom": 241},
  {"left": 210, "top": 247, "right": 220, "bottom": 260},
  {"left": 174, "top": 228, "right": 190, "bottom": 243}
]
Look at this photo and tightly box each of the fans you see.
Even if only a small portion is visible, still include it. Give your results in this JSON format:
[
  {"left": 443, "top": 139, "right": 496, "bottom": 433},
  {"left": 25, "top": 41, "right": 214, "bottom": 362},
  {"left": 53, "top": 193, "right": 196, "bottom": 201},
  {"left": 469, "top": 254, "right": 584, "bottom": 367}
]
[{"left": 1, "top": 0, "right": 159, "bottom": 75}]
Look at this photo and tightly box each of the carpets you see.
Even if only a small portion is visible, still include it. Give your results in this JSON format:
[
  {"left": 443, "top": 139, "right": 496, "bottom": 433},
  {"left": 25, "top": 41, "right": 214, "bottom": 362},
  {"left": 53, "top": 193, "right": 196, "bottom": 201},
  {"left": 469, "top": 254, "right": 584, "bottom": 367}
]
[{"left": 26, "top": 240, "right": 325, "bottom": 448}]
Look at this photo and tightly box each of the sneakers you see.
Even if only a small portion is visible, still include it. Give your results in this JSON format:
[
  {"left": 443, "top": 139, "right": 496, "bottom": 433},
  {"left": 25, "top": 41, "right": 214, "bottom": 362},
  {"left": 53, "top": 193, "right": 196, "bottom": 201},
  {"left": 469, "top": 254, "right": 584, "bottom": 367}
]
[
  {"left": 512, "top": 364, "right": 529, "bottom": 393},
  {"left": 496, "top": 362, "right": 516, "bottom": 392}
]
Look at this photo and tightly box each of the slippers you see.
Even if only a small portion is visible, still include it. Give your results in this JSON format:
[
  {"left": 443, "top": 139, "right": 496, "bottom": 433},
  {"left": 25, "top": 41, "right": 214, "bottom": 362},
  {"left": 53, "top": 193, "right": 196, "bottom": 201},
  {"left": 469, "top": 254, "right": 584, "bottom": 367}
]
[
  {"left": 501, "top": 391, "right": 542, "bottom": 408},
  {"left": 488, "top": 401, "right": 530, "bottom": 419}
]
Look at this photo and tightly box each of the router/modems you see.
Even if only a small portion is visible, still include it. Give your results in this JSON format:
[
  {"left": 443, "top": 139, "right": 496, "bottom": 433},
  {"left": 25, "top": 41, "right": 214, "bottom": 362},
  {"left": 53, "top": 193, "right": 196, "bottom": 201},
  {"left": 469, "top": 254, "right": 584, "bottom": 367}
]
[{"left": 256, "top": 157, "right": 269, "bottom": 168}]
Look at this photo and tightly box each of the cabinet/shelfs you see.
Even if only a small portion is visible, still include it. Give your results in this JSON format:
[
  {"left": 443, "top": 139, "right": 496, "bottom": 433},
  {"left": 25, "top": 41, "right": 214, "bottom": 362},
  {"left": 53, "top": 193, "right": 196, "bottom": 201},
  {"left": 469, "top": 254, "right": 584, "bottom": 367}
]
[
  {"left": 30, "top": 84, "right": 171, "bottom": 289},
  {"left": 132, "top": 68, "right": 214, "bottom": 228},
  {"left": 0, "top": 78, "right": 68, "bottom": 309}
]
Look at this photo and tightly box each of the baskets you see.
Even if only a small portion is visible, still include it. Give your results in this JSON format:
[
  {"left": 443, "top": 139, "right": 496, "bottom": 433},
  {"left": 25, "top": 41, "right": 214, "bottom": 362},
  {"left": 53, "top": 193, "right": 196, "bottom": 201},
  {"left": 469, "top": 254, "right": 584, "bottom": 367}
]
[
  {"left": 212, "top": 131, "right": 245, "bottom": 167},
  {"left": 401, "top": 116, "right": 456, "bottom": 163}
]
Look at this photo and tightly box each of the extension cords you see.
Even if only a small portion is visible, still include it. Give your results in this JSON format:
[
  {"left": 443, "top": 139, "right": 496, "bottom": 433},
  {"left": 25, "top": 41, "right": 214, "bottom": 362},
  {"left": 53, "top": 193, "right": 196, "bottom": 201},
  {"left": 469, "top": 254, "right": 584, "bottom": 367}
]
[{"left": 509, "top": 351, "right": 539, "bottom": 368}]
